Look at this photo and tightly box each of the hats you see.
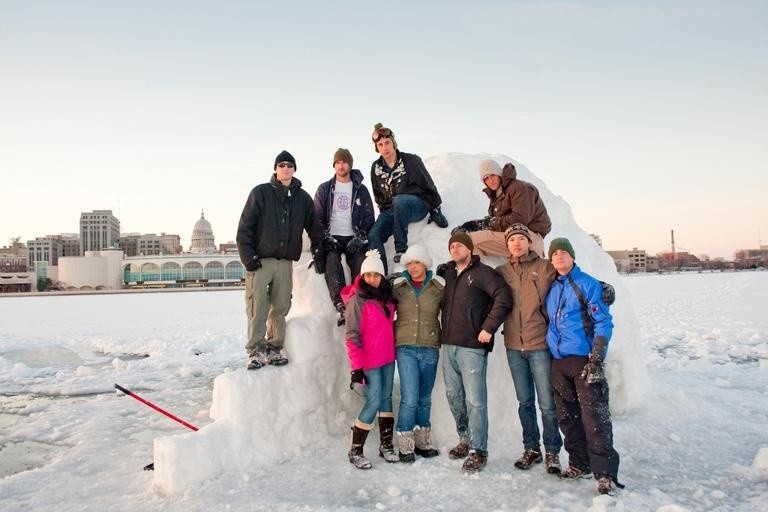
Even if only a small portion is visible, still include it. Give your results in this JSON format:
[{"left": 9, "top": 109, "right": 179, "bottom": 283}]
[
  {"left": 333, "top": 148, "right": 353, "bottom": 168},
  {"left": 547, "top": 237, "right": 575, "bottom": 263},
  {"left": 360, "top": 249, "right": 385, "bottom": 280},
  {"left": 372, "top": 123, "right": 394, "bottom": 143},
  {"left": 504, "top": 222, "right": 532, "bottom": 248},
  {"left": 448, "top": 233, "right": 473, "bottom": 255},
  {"left": 427, "top": 206, "right": 448, "bottom": 229},
  {"left": 479, "top": 159, "right": 502, "bottom": 186},
  {"left": 579, "top": 362, "right": 606, "bottom": 385},
  {"left": 274, "top": 150, "right": 296, "bottom": 173},
  {"left": 399, "top": 243, "right": 432, "bottom": 269}
]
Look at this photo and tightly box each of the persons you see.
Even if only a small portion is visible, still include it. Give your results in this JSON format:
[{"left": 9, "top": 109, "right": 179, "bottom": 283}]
[
  {"left": 385, "top": 244, "right": 447, "bottom": 465},
  {"left": 496, "top": 223, "right": 616, "bottom": 474},
  {"left": 340, "top": 249, "right": 401, "bottom": 470},
  {"left": 367, "top": 123, "right": 448, "bottom": 277},
  {"left": 236, "top": 149, "right": 323, "bottom": 369},
  {"left": 436, "top": 231, "right": 513, "bottom": 471},
  {"left": 546, "top": 237, "right": 625, "bottom": 493},
  {"left": 452, "top": 160, "right": 551, "bottom": 260},
  {"left": 311, "top": 147, "right": 375, "bottom": 326}
]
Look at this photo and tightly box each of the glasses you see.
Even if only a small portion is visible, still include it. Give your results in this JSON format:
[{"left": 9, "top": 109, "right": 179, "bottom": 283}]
[{"left": 276, "top": 160, "right": 296, "bottom": 168}]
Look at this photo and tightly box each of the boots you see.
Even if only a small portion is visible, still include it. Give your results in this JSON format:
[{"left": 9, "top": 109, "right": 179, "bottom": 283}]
[
  {"left": 349, "top": 418, "right": 374, "bottom": 470},
  {"left": 376, "top": 409, "right": 400, "bottom": 463}
]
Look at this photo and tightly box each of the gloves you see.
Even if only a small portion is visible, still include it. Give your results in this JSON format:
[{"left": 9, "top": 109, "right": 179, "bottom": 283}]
[
  {"left": 326, "top": 237, "right": 341, "bottom": 252},
  {"left": 246, "top": 258, "right": 262, "bottom": 272},
  {"left": 348, "top": 367, "right": 369, "bottom": 399},
  {"left": 344, "top": 237, "right": 364, "bottom": 260},
  {"left": 599, "top": 278, "right": 615, "bottom": 307},
  {"left": 458, "top": 219, "right": 481, "bottom": 235},
  {"left": 313, "top": 251, "right": 325, "bottom": 273}
]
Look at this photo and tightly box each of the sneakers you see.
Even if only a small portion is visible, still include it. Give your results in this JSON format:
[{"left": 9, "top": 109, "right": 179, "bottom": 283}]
[
  {"left": 397, "top": 449, "right": 416, "bottom": 464},
  {"left": 413, "top": 443, "right": 439, "bottom": 458},
  {"left": 461, "top": 453, "right": 487, "bottom": 473},
  {"left": 561, "top": 465, "right": 593, "bottom": 482},
  {"left": 544, "top": 451, "right": 562, "bottom": 475},
  {"left": 247, "top": 348, "right": 289, "bottom": 371},
  {"left": 513, "top": 448, "right": 543, "bottom": 470},
  {"left": 597, "top": 477, "right": 616, "bottom": 496},
  {"left": 335, "top": 302, "right": 348, "bottom": 328},
  {"left": 448, "top": 443, "right": 469, "bottom": 460}
]
